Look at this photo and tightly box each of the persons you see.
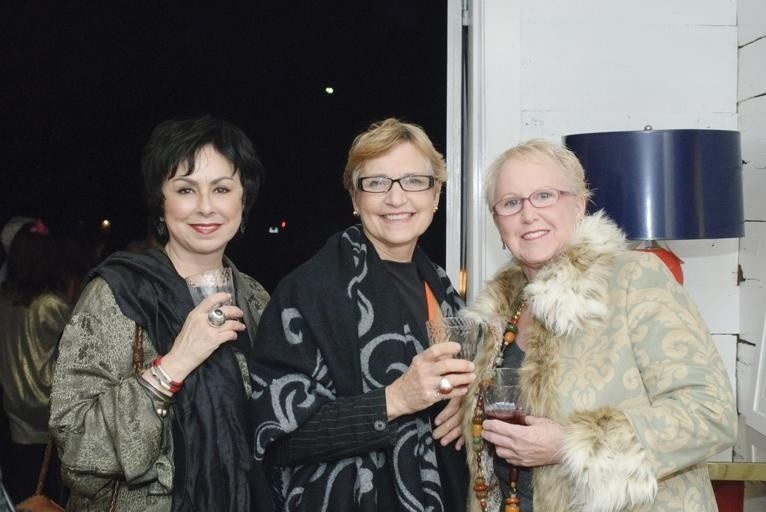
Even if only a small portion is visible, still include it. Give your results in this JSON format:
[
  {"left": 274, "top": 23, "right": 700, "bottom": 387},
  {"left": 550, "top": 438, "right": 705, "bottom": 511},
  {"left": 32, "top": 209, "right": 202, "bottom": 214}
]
[
  {"left": 0, "top": 215, "right": 38, "bottom": 284},
  {"left": 247, "top": 117, "right": 478, "bottom": 511},
  {"left": 462, "top": 139, "right": 739, "bottom": 511},
  {"left": 1, "top": 222, "right": 73, "bottom": 509},
  {"left": 46, "top": 112, "right": 271, "bottom": 511}
]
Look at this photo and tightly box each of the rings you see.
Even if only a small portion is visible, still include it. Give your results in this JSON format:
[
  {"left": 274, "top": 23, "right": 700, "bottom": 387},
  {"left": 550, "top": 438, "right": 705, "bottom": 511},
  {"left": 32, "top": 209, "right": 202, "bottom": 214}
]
[
  {"left": 437, "top": 377, "right": 453, "bottom": 395},
  {"left": 208, "top": 305, "right": 226, "bottom": 326}
]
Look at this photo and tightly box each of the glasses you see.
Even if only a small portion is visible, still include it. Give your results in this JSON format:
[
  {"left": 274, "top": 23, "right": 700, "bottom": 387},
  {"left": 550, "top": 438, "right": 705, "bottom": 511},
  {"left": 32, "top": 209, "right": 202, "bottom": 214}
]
[
  {"left": 355, "top": 172, "right": 440, "bottom": 194},
  {"left": 492, "top": 187, "right": 583, "bottom": 215}
]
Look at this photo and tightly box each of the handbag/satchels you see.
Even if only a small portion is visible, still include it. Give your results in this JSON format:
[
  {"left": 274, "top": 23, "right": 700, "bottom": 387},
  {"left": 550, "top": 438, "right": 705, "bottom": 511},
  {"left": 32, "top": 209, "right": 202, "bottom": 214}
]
[{"left": 15, "top": 493, "right": 68, "bottom": 512}]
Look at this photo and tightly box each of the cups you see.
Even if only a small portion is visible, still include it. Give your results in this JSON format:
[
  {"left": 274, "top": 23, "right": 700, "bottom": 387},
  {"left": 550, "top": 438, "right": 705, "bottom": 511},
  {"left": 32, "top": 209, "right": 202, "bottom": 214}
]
[
  {"left": 425, "top": 316, "right": 475, "bottom": 369},
  {"left": 482, "top": 367, "right": 525, "bottom": 429},
  {"left": 185, "top": 264, "right": 236, "bottom": 317}
]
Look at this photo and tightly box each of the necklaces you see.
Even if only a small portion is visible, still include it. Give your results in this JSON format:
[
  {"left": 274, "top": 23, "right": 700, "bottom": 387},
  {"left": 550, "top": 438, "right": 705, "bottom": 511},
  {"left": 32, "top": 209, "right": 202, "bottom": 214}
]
[{"left": 471, "top": 299, "right": 532, "bottom": 512}]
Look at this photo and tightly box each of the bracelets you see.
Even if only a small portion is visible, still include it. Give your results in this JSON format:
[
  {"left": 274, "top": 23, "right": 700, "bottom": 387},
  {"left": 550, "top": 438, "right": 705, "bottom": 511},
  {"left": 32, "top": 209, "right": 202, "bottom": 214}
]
[
  {"left": 154, "top": 352, "right": 184, "bottom": 388},
  {"left": 147, "top": 358, "right": 182, "bottom": 394}
]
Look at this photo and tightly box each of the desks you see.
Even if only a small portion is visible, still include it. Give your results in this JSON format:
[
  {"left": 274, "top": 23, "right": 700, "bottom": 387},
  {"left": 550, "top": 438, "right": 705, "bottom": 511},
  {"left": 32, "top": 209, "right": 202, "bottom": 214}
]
[{"left": 691, "top": 419, "right": 764, "bottom": 511}]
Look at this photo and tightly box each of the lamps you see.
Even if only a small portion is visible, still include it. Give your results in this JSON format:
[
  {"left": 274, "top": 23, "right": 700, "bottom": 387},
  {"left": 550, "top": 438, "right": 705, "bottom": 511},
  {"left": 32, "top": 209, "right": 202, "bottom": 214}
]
[{"left": 559, "top": 121, "right": 746, "bottom": 289}]
